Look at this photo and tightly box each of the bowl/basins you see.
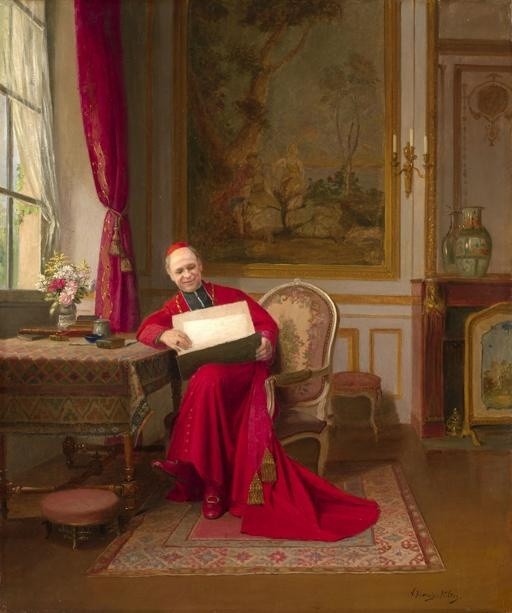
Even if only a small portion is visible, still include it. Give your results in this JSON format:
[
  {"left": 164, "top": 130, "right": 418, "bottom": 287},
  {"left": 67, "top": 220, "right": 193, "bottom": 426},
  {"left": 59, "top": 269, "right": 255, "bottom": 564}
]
[{"left": 85, "top": 334, "right": 103, "bottom": 343}]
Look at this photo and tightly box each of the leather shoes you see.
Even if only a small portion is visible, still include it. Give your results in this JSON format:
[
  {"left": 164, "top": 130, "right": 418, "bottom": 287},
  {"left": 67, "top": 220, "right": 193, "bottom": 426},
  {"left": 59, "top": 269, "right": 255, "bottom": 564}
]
[
  {"left": 202, "top": 490, "right": 224, "bottom": 520},
  {"left": 147, "top": 455, "right": 194, "bottom": 485}
]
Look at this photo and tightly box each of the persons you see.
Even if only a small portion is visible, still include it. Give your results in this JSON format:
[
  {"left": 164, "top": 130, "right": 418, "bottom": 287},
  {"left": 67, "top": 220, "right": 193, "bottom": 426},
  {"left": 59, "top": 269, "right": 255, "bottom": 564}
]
[{"left": 136, "top": 242, "right": 383, "bottom": 550}]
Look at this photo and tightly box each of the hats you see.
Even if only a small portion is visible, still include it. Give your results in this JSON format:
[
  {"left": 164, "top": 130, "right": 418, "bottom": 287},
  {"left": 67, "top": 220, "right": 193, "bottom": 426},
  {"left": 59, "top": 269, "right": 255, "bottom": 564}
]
[{"left": 165, "top": 241, "right": 191, "bottom": 256}]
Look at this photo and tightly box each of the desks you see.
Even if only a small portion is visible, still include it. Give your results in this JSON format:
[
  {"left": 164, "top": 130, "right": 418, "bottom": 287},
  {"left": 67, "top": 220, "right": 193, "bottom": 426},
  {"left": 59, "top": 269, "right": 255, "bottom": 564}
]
[{"left": 0, "top": 331, "right": 182, "bottom": 516}]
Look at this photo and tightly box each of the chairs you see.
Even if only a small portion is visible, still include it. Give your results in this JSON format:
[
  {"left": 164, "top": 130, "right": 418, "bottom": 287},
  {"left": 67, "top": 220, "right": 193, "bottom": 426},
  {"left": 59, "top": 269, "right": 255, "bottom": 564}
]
[{"left": 256, "top": 278, "right": 340, "bottom": 477}]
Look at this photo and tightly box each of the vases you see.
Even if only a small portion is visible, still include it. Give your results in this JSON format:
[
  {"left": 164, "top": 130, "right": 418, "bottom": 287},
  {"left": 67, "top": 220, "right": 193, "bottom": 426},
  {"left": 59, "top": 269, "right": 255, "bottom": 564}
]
[
  {"left": 35, "top": 250, "right": 96, "bottom": 318},
  {"left": 441, "top": 211, "right": 462, "bottom": 274},
  {"left": 452, "top": 206, "right": 492, "bottom": 276},
  {"left": 57, "top": 313, "right": 77, "bottom": 330}
]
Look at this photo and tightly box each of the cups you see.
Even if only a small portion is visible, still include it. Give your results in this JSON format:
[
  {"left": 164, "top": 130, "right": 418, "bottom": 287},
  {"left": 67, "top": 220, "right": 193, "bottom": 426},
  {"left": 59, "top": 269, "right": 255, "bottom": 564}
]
[{"left": 94, "top": 320, "right": 110, "bottom": 335}]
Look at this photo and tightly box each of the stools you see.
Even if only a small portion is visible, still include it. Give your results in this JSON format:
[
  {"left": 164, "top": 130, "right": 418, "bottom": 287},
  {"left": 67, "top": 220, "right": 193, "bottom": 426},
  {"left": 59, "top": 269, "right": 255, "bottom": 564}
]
[
  {"left": 328, "top": 371, "right": 382, "bottom": 435},
  {"left": 40, "top": 488, "right": 122, "bottom": 550}
]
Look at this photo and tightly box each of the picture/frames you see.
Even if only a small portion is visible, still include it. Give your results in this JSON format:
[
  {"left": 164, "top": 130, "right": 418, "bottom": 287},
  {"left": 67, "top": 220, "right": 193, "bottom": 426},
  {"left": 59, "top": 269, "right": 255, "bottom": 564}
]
[{"left": 172, "top": 0, "right": 400, "bottom": 283}]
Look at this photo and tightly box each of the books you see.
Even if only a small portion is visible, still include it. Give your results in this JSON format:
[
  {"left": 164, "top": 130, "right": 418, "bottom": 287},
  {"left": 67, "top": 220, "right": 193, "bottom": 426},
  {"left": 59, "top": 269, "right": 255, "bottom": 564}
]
[{"left": 168, "top": 300, "right": 263, "bottom": 375}]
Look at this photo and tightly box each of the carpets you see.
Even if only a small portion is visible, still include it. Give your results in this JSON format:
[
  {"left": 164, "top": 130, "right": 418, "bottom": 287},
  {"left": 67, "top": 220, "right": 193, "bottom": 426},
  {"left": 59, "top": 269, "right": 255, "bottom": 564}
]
[{"left": 81, "top": 458, "right": 447, "bottom": 577}]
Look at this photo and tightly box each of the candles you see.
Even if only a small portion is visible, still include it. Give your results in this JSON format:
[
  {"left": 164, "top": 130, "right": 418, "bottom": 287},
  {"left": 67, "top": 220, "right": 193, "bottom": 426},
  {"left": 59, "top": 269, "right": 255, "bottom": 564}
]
[
  {"left": 391, "top": 134, "right": 398, "bottom": 153},
  {"left": 423, "top": 135, "right": 428, "bottom": 154},
  {"left": 409, "top": 127, "right": 415, "bottom": 146}
]
